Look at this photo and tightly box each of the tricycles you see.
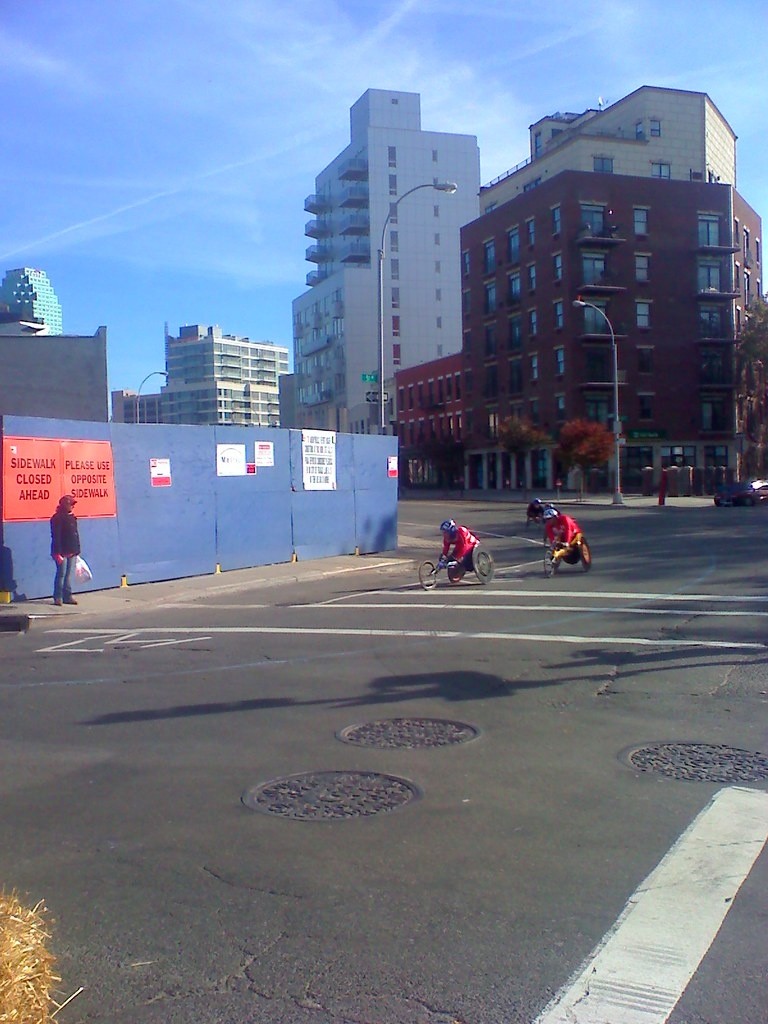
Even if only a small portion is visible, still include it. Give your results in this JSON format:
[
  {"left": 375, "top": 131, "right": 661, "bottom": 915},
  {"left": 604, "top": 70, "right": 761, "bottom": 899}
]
[
  {"left": 418, "top": 543, "right": 494, "bottom": 592},
  {"left": 544, "top": 529, "right": 592, "bottom": 578}
]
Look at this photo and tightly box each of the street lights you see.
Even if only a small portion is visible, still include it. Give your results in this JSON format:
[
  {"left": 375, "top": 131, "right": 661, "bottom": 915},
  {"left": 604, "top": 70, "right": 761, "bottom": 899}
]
[
  {"left": 573, "top": 300, "right": 625, "bottom": 504},
  {"left": 134, "top": 370, "right": 170, "bottom": 424},
  {"left": 372, "top": 180, "right": 458, "bottom": 431}
]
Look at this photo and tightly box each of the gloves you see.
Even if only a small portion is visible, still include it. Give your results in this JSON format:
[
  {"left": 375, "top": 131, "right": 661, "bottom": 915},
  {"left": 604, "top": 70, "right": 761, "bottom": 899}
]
[
  {"left": 552, "top": 540, "right": 568, "bottom": 547},
  {"left": 447, "top": 557, "right": 454, "bottom": 564},
  {"left": 439, "top": 554, "right": 444, "bottom": 561}
]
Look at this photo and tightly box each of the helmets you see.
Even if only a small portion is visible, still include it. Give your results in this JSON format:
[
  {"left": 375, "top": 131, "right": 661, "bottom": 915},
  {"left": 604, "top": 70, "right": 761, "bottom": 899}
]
[
  {"left": 543, "top": 509, "right": 557, "bottom": 519},
  {"left": 545, "top": 504, "right": 554, "bottom": 510},
  {"left": 533, "top": 499, "right": 542, "bottom": 504},
  {"left": 440, "top": 519, "right": 456, "bottom": 535}
]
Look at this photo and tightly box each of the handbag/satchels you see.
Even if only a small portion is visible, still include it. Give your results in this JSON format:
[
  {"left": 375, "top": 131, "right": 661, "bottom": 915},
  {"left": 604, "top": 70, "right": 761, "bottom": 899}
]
[{"left": 74, "top": 555, "right": 92, "bottom": 584}]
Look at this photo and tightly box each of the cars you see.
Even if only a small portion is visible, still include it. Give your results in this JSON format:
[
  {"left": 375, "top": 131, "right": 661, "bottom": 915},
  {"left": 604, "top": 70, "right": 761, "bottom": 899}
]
[{"left": 713, "top": 478, "right": 768, "bottom": 508}]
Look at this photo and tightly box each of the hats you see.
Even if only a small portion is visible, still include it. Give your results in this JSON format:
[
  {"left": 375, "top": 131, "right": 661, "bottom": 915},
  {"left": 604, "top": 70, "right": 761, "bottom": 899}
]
[{"left": 61, "top": 496, "right": 77, "bottom": 508}]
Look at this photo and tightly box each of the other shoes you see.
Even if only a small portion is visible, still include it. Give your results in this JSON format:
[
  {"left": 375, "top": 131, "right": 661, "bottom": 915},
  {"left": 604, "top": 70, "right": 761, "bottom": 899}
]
[
  {"left": 54, "top": 599, "right": 62, "bottom": 605},
  {"left": 64, "top": 598, "right": 77, "bottom": 604}
]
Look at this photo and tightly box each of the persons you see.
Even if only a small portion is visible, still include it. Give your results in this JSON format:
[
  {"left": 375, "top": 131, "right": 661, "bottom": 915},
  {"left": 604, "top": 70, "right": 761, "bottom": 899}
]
[
  {"left": 527, "top": 499, "right": 545, "bottom": 521},
  {"left": 50, "top": 495, "right": 82, "bottom": 605},
  {"left": 439, "top": 518, "right": 480, "bottom": 573},
  {"left": 543, "top": 509, "right": 582, "bottom": 550}
]
